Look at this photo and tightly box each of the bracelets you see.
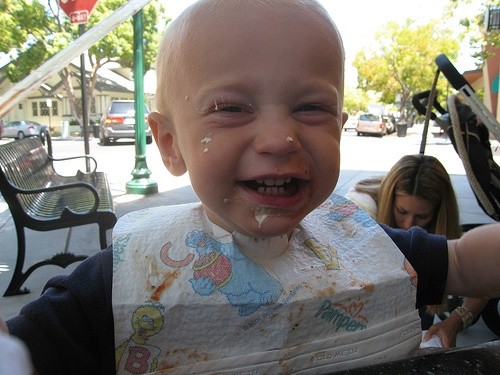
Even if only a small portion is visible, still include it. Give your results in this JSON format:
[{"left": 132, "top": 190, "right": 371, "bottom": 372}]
[{"left": 455, "top": 305, "right": 475, "bottom": 330}]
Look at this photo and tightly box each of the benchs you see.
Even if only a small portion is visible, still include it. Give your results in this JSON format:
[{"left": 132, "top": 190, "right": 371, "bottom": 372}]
[{"left": 0, "top": 136, "right": 117, "bottom": 297}]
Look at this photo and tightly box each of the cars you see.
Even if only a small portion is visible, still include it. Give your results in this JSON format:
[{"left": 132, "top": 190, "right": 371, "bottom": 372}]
[
  {"left": 342, "top": 116, "right": 358, "bottom": 131},
  {"left": 99, "top": 100, "right": 152, "bottom": 145},
  {"left": 0, "top": 120, "right": 50, "bottom": 140},
  {"left": 355, "top": 112, "right": 396, "bottom": 137}
]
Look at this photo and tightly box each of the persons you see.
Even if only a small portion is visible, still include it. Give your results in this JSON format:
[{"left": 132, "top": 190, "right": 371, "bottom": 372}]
[
  {"left": 345, "top": 155, "right": 488, "bottom": 348},
  {"left": 0, "top": 0, "right": 500, "bottom": 375}
]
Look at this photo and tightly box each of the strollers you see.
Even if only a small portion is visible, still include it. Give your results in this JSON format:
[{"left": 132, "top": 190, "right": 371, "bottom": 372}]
[{"left": 410, "top": 53, "right": 500, "bottom": 223}]
